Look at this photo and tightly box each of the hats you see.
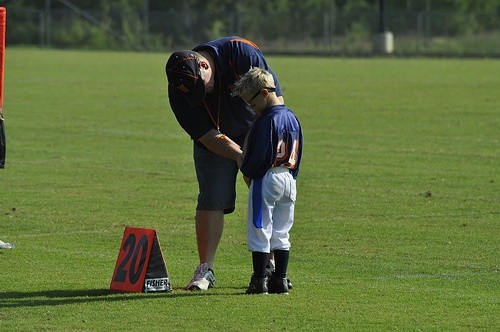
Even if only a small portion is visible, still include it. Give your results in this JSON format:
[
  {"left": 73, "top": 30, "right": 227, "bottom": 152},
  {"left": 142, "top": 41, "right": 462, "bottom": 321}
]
[{"left": 165, "top": 50, "right": 205, "bottom": 107}]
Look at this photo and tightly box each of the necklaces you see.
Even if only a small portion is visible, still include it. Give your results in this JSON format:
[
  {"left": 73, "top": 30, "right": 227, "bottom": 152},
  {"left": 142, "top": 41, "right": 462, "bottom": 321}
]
[{"left": 203, "top": 49, "right": 222, "bottom": 134}]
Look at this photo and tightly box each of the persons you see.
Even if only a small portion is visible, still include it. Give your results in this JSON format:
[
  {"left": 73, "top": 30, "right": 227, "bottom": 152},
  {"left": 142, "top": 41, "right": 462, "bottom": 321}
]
[
  {"left": 230, "top": 67, "right": 303, "bottom": 294},
  {"left": 166, "top": 36, "right": 285, "bottom": 290}
]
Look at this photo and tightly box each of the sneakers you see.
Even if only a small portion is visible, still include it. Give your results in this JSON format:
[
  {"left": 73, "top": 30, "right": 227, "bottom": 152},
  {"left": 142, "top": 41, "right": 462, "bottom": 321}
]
[
  {"left": 264, "top": 259, "right": 293, "bottom": 289},
  {"left": 187, "top": 263, "right": 215, "bottom": 292},
  {"left": 246, "top": 275, "right": 269, "bottom": 294},
  {"left": 269, "top": 275, "right": 288, "bottom": 294}
]
